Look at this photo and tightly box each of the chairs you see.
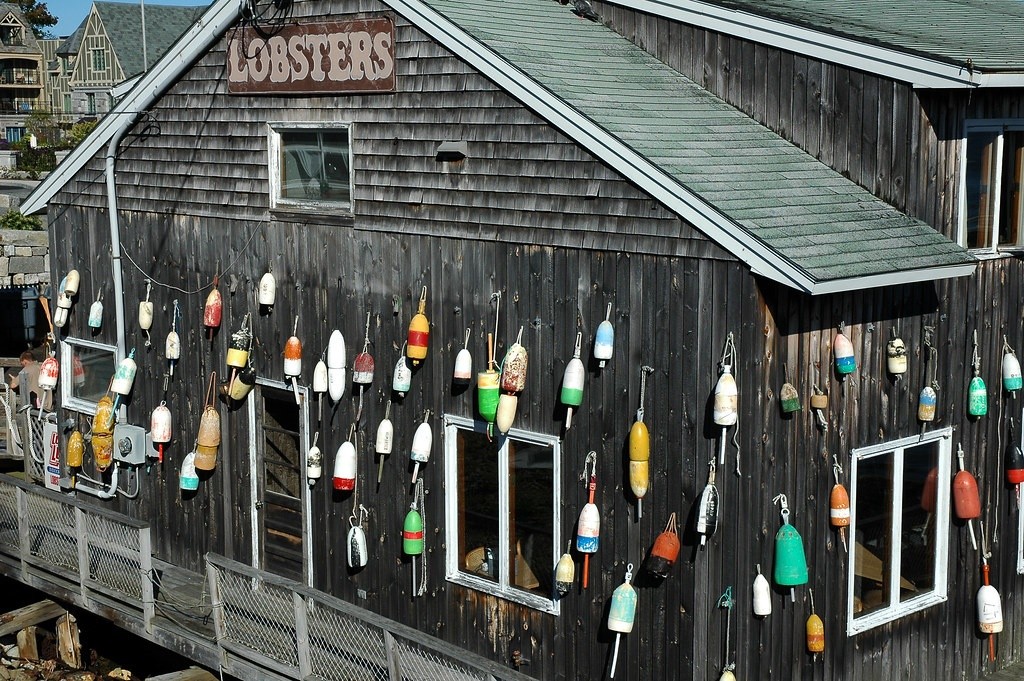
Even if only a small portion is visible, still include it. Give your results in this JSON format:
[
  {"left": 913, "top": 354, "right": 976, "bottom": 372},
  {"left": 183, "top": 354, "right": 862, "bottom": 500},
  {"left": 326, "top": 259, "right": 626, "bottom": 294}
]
[{"left": 15, "top": 73, "right": 25, "bottom": 84}]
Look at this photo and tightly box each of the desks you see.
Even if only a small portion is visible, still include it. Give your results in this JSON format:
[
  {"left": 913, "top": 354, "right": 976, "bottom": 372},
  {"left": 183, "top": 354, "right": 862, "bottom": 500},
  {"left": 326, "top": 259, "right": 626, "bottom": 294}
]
[{"left": 465, "top": 468, "right": 553, "bottom": 565}]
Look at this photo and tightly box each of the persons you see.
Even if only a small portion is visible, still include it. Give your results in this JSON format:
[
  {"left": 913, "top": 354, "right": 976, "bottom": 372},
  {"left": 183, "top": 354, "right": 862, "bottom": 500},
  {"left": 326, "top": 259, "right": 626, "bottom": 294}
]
[{"left": 8, "top": 352, "right": 53, "bottom": 413}]
